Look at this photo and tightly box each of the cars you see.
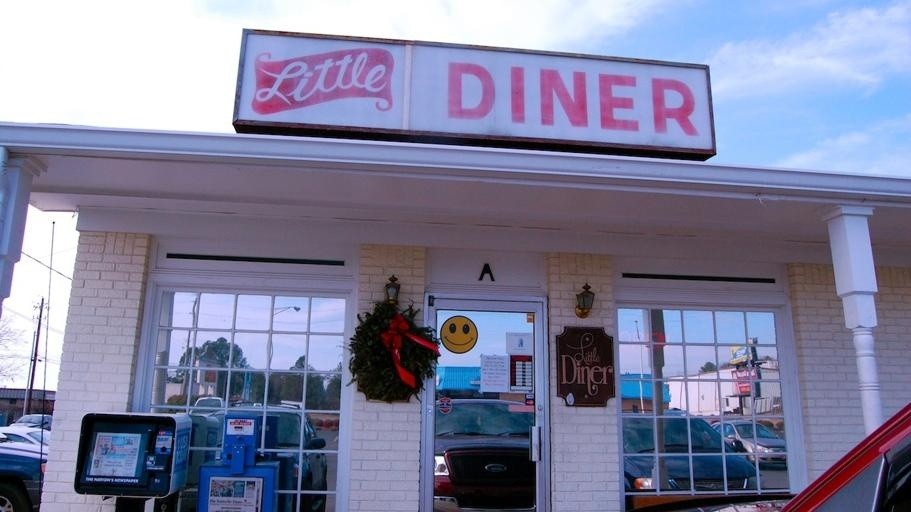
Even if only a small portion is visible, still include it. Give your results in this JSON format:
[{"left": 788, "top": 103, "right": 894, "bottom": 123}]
[
  {"left": 0, "top": 414, "right": 52, "bottom": 512},
  {"left": 434, "top": 399, "right": 534, "bottom": 512},
  {"left": 190, "top": 396, "right": 327, "bottom": 512},
  {"left": 779, "top": 403, "right": 911, "bottom": 512},
  {"left": 622, "top": 408, "right": 787, "bottom": 492}
]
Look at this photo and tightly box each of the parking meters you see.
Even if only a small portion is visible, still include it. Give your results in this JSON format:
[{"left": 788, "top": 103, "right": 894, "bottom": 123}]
[{"left": 74, "top": 412, "right": 192, "bottom": 499}]
